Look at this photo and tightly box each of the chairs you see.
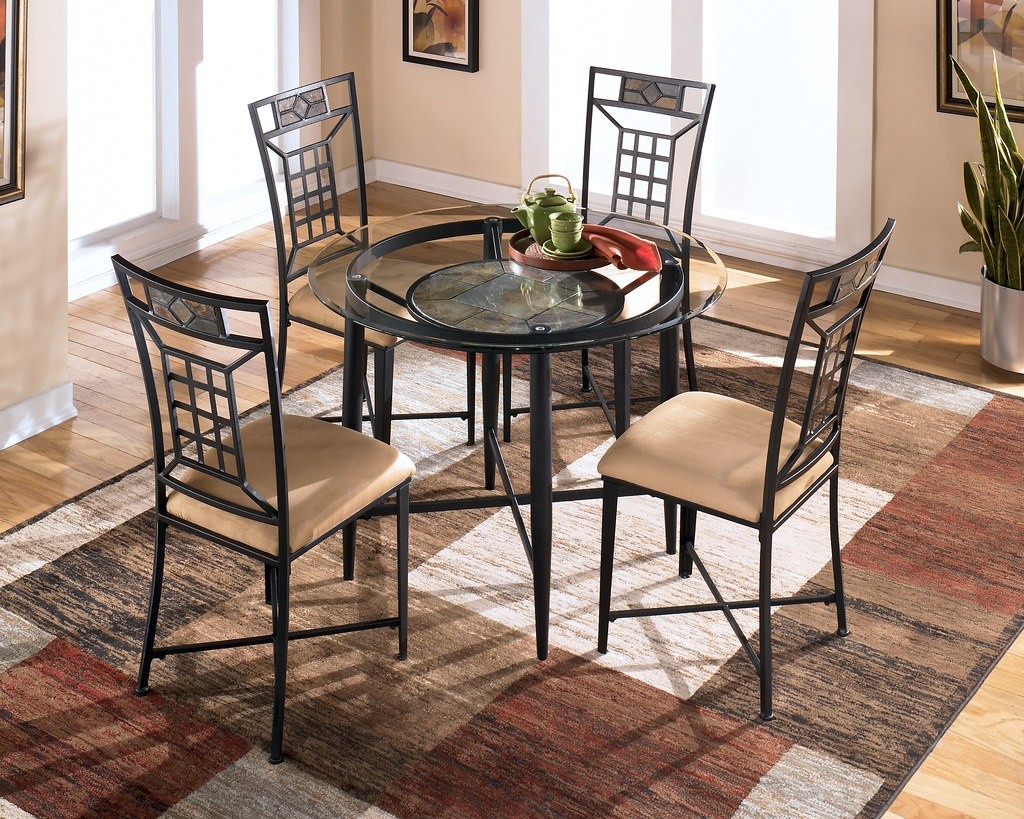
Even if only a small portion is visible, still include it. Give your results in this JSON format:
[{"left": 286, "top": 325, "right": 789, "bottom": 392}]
[
  {"left": 112, "top": 254, "right": 417, "bottom": 764},
  {"left": 596, "top": 219, "right": 897, "bottom": 722},
  {"left": 248, "top": 73, "right": 474, "bottom": 451},
  {"left": 504, "top": 66, "right": 717, "bottom": 445}
]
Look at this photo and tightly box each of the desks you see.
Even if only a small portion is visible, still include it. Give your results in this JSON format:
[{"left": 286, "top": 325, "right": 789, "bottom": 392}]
[{"left": 308, "top": 205, "right": 728, "bottom": 662}]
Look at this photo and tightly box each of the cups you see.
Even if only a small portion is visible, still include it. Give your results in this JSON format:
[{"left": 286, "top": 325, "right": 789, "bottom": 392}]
[
  {"left": 549, "top": 211, "right": 584, "bottom": 232},
  {"left": 548, "top": 224, "right": 584, "bottom": 252}
]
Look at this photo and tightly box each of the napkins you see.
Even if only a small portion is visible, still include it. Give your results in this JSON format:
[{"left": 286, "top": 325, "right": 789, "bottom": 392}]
[{"left": 580, "top": 225, "right": 661, "bottom": 274}]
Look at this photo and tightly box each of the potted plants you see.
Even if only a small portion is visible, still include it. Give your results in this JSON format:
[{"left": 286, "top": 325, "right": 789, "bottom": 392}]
[{"left": 950, "top": 51, "right": 1024, "bottom": 376}]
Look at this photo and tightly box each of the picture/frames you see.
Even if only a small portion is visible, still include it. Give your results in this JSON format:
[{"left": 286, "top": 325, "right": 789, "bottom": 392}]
[
  {"left": 0, "top": 0, "right": 27, "bottom": 207},
  {"left": 401, "top": 0, "right": 481, "bottom": 74},
  {"left": 936, "top": 0, "right": 1024, "bottom": 122}
]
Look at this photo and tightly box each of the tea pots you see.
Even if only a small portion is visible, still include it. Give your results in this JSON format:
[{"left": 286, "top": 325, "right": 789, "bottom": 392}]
[{"left": 511, "top": 174, "right": 575, "bottom": 248}]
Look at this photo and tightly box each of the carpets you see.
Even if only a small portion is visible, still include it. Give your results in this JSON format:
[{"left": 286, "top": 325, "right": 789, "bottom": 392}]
[{"left": 2, "top": 319, "right": 1024, "bottom": 819}]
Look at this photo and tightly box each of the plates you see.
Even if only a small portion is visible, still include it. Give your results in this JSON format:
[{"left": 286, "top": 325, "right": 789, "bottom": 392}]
[
  {"left": 541, "top": 247, "right": 592, "bottom": 260},
  {"left": 543, "top": 238, "right": 593, "bottom": 256}
]
